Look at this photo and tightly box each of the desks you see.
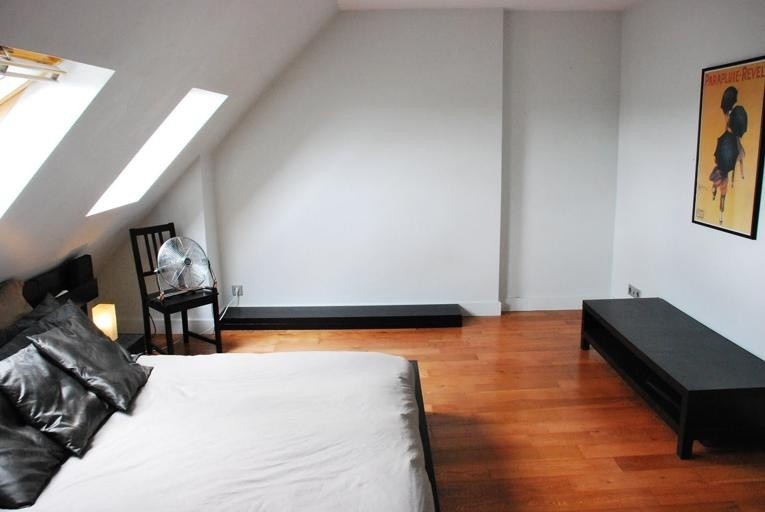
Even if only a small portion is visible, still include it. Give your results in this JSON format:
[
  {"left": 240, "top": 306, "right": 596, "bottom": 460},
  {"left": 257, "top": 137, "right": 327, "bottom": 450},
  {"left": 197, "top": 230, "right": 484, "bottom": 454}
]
[{"left": 581, "top": 297, "right": 765, "bottom": 460}]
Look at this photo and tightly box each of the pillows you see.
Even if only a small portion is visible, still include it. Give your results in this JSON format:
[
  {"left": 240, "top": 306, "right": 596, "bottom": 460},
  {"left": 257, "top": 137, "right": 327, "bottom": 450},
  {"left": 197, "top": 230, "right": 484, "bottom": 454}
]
[
  {"left": 26, "top": 311, "right": 154, "bottom": 413},
  {"left": 0, "top": 343, "right": 116, "bottom": 460},
  {"left": 1, "top": 278, "right": 34, "bottom": 329},
  {"left": 0, "top": 298, "right": 133, "bottom": 509},
  {"left": 1, "top": 292, "right": 64, "bottom": 348}
]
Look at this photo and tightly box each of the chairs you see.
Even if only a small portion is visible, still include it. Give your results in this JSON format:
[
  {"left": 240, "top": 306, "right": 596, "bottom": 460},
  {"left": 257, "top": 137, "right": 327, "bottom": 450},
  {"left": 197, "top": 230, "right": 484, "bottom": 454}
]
[{"left": 129, "top": 222, "right": 223, "bottom": 353}]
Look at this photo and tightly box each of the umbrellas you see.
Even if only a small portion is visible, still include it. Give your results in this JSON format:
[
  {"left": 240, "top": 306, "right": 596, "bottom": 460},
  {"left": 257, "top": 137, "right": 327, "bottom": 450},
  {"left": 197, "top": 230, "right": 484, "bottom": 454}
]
[
  {"left": 714, "top": 129, "right": 740, "bottom": 173},
  {"left": 729, "top": 105, "right": 748, "bottom": 137},
  {"left": 720, "top": 86, "right": 737, "bottom": 114}
]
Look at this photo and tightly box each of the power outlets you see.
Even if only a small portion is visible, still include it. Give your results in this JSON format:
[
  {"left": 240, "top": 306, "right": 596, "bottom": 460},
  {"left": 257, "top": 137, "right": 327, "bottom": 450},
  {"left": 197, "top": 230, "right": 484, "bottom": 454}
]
[
  {"left": 232, "top": 285, "right": 243, "bottom": 297},
  {"left": 628, "top": 284, "right": 640, "bottom": 298}
]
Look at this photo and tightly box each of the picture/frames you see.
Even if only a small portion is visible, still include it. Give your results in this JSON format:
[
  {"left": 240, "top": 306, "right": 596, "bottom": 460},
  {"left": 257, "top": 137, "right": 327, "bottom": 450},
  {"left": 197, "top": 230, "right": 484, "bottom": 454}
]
[{"left": 691, "top": 56, "right": 765, "bottom": 240}]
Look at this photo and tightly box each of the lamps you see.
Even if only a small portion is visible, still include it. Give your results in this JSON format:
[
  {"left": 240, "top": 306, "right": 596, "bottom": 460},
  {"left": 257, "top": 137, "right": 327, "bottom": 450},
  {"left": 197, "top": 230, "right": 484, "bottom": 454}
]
[{"left": 92, "top": 303, "right": 119, "bottom": 342}]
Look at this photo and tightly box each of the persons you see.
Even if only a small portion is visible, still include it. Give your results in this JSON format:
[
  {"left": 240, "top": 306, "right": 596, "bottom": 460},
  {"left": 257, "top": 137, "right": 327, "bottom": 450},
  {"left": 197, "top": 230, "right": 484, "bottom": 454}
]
[
  {"left": 726, "top": 125, "right": 746, "bottom": 187},
  {"left": 709, "top": 166, "right": 729, "bottom": 226}
]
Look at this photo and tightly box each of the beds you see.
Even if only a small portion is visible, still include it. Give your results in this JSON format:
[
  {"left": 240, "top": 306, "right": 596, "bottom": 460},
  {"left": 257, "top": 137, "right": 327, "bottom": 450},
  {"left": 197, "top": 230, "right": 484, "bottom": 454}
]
[{"left": 0, "top": 255, "right": 441, "bottom": 512}]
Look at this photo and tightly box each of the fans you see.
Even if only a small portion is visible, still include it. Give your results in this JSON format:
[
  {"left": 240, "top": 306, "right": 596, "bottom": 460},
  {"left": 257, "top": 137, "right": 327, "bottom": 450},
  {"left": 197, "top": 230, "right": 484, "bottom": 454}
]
[{"left": 154, "top": 237, "right": 217, "bottom": 302}]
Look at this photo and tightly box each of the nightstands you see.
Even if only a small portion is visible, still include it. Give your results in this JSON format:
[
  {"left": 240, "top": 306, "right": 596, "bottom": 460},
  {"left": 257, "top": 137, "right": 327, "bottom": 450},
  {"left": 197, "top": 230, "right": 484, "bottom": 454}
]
[{"left": 117, "top": 333, "right": 144, "bottom": 353}]
